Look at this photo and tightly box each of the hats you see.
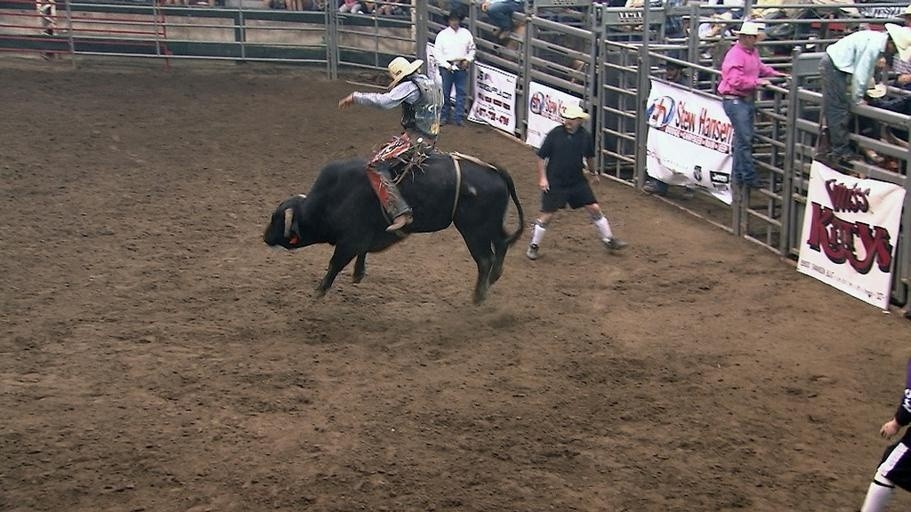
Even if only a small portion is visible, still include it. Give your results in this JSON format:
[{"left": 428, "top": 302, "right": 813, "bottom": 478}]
[
  {"left": 387, "top": 56, "right": 424, "bottom": 92},
  {"left": 732, "top": 22, "right": 766, "bottom": 40},
  {"left": 712, "top": 11, "right": 731, "bottom": 21},
  {"left": 560, "top": 104, "right": 589, "bottom": 121},
  {"left": 884, "top": 22, "right": 911, "bottom": 63},
  {"left": 896, "top": 6, "right": 911, "bottom": 18},
  {"left": 443, "top": 10, "right": 465, "bottom": 21}
]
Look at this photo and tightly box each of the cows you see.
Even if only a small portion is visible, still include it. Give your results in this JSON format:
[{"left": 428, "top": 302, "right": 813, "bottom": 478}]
[{"left": 262, "top": 149, "right": 525, "bottom": 306}]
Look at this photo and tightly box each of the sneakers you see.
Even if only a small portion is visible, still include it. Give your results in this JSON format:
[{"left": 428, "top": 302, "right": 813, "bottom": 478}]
[
  {"left": 681, "top": 188, "right": 693, "bottom": 200},
  {"left": 385, "top": 214, "right": 413, "bottom": 231},
  {"left": 440, "top": 121, "right": 446, "bottom": 125},
  {"left": 526, "top": 242, "right": 540, "bottom": 259},
  {"left": 458, "top": 122, "right": 466, "bottom": 126},
  {"left": 606, "top": 238, "right": 628, "bottom": 249},
  {"left": 644, "top": 186, "right": 664, "bottom": 195}
]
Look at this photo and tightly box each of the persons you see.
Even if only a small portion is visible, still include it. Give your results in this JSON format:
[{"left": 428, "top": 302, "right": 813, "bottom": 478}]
[
  {"left": 820, "top": 22, "right": 911, "bottom": 174},
  {"left": 718, "top": 21, "right": 794, "bottom": 191},
  {"left": 481, "top": 1, "right": 527, "bottom": 44},
  {"left": 604, "top": 0, "right": 874, "bottom": 65},
  {"left": 337, "top": 1, "right": 412, "bottom": 17},
  {"left": 526, "top": 107, "right": 630, "bottom": 261},
  {"left": 433, "top": 8, "right": 477, "bottom": 130},
  {"left": 36, "top": 1, "right": 61, "bottom": 62},
  {"left": 859, "top": 357, "right": 911, "bottom": 510},
  {"left": 338, "top": 54, "right": 448, "bottom": 235}
]
